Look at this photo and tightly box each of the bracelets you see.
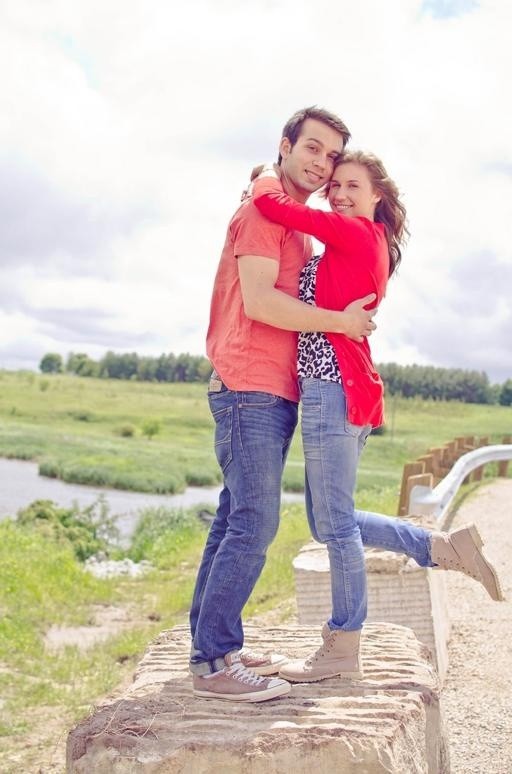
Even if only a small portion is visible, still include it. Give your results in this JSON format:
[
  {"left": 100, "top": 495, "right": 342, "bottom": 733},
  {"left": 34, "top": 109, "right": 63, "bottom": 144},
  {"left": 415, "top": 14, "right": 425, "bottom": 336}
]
[{"left": 262, "top": 168, "right": 280, "bottom": 172}]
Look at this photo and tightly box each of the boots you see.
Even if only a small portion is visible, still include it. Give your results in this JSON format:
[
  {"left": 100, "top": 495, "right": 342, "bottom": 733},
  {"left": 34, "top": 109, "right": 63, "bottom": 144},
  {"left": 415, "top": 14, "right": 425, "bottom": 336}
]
[
  {"left": 277, "top": 622, "right": 363, "bottom": 686},
  {"left": 430, "top": 522, "right": 502, "bottom": 602}
]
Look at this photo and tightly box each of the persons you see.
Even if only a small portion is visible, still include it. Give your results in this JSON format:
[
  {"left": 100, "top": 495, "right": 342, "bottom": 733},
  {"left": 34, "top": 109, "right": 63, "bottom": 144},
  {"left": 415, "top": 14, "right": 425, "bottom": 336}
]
[
  {"left": 249, "top": 148, "right": 503, "bottom": 683},
  {"left": 189, "top": 106, "right": 378, "bottom": 703}
]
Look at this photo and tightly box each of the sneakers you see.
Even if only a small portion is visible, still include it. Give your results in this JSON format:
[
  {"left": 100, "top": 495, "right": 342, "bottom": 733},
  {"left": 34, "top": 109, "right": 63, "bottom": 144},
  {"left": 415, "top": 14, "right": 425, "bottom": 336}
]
[
  {"left": 238, "top": 646, "right": 290, "bottom": 676},
  {"left": 192, "top": 662, "right": 293, "bottom": 703}
]
